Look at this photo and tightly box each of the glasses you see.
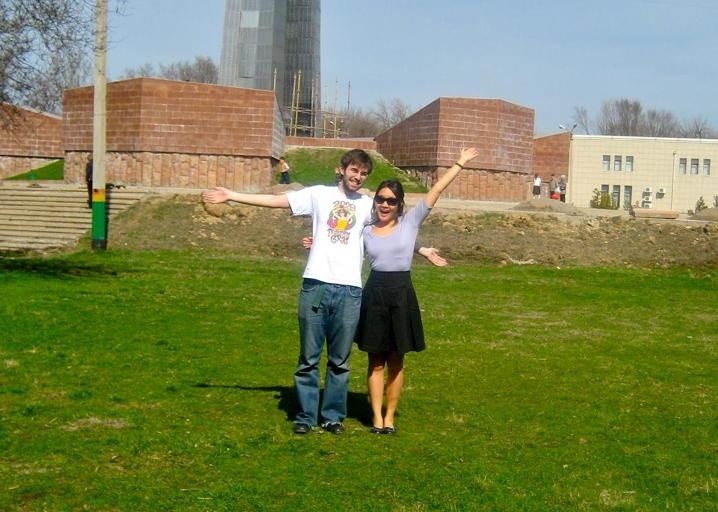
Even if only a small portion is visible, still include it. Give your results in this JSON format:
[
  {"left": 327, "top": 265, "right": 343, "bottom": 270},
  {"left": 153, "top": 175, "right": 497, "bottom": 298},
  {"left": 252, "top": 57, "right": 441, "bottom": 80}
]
[{"left": 374, "top": 195, "right": 398, "bottom": 207}]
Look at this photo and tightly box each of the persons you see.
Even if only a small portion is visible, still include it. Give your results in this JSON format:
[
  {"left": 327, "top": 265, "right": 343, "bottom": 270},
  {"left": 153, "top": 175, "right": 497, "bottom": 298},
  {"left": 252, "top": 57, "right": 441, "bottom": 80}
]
[
  {"left": 85, "top": 152, "right": 93, "bottom": 209},
  {"left": 549, "top": 174, "right": 567, "bottom": 202},
  {"left": 301, "top": 146, "right": 478, "bottom": 434},
  {"left": 201, "top": 151, "right": 449, "bottom": 435},
  {"left": 278, "top": 156, "right": 290, "bottom": 184},
  {"left": 532, "top": 172, "right": 541, "bottom": 200}
]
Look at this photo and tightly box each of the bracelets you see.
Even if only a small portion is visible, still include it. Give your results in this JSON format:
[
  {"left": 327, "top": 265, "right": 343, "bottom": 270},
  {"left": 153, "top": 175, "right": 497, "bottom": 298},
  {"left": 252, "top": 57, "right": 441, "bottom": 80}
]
[{"left": 454, "top": 162, "right": 464, "bottom": 170}]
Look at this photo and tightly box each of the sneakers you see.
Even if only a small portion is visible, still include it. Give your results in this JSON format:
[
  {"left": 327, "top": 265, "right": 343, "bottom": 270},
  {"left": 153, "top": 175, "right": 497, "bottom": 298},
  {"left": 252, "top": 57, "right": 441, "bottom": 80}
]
[
  {"left": 295, "top": 422, "right": 310, "bottom": 434},
  {"left": 324, "top": 424, "right": 344, "bottom": 434}
]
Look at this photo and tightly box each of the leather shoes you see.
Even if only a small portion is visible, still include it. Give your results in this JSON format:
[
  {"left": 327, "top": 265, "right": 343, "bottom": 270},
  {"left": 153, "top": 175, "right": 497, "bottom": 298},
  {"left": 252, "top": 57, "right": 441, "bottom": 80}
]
[{"left": 373, "top": 426, "right": 396, "bottom": 434}]
